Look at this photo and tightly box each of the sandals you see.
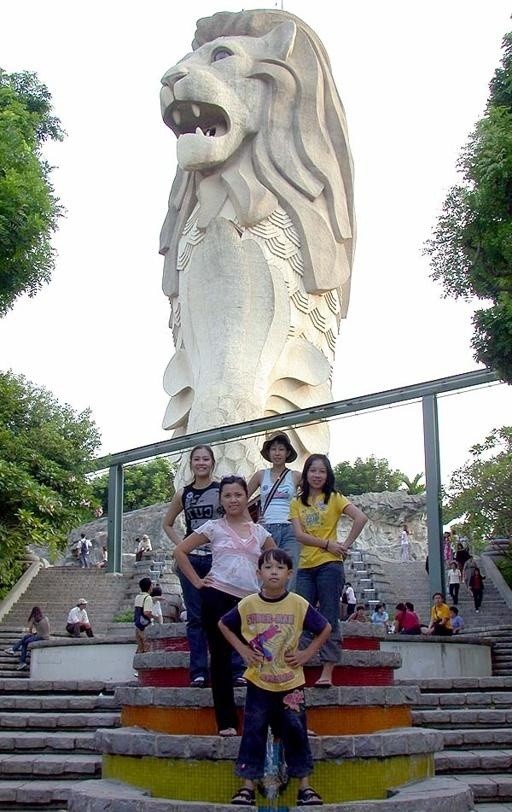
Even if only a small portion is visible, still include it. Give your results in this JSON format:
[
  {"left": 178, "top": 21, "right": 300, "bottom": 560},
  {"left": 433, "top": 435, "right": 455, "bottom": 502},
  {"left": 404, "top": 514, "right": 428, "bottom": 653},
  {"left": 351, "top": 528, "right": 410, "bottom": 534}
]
[
  {"left": 296, "top": 788, "right": 323, "bottom": 805},
  {"left": 306, "top": 730, "right": 317, "bottom": 735},
  {"left": 231, "top": 788, "right": 257, "bottom": 805},
  {"left": 315, "top": 680, "right": 331, "bottom": 686},
  {"left": 220, "top": 728, "right": 236, "bottom": 736}
]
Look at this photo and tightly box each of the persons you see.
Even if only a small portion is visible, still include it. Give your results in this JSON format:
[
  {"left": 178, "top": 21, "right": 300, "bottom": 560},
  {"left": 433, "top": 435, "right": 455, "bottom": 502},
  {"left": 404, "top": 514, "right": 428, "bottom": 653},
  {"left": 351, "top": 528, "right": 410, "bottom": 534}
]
[
  {"left": 174, "top": 476, "right": 279, "bottom": 736},
  {"left": 347, "top": 604, "right": 367, "bottom": 622},
  {"left": 372, "top": 602, "right": 390, "bottom": 633},
  {"left": 457, "top": 542, "right": 469, "bottom": 582},
  {"left": 66, "top": 598, "right": 95, "bottom": 638},
  {"left": 135, "top": 537, "right": 140, "bottom": 553},
  {"left": 96, "top": 505, "right": 103, "bottom": 519},
  {"left": 137, "top": 534, "right": 152, "bottom": 554},
  {"left": 164, "top": 445, "right": 248, "bottom": 688},
  {"left": 392, "top": 602, "right": 422, "bottom": 635},
  {"left": 78, "top": 534, "right": 93, "bottom": 568},
  {"left": 468, "top": 568, "right": 485, "bottom": 615},
  {"left": 405, "top": 602, "right": 420, "bottom": 628},
  {"left": 344, "top": 581, "right": 357, "bottom": 619},
  {"left": 134, "top": 578, "right": 152, "bottom": 678},
  {"left": 5, "top": 607, "right": 51, "bottom": 670},
  {"left": 420, "top": 593, "right": 451, "bottom": 635},
  {"left": 447, "top": 562, "right": 461, "bottom": 606},
  {"left": 444, "top": 531, "right": 451, "bottom": 565},
  {"left": 450, "top": 606, "right": 464, "bottom": 634},
  {"left": 401, "top": 524, "right": 410, "bottom": 563},
  {"left": 463, "top": 556, "right": 477, "bottom": 597},
  {"left": 97, "top": 546, "right": 108, "bottom": 570},
  {"left": 247, "top": 431, "right": 302, "bottom": 595},
  {"left": 288, "top": 453, "right": 367, "bottom": 687},
  {"left": 219, "top": 549, "right": 332, "bottom": 806},
  {"left": 451, "top": 530, "right": 458, "bottom": 541},
  {"left": 150, "top": 587, "right": 164, "bottom": 624}
]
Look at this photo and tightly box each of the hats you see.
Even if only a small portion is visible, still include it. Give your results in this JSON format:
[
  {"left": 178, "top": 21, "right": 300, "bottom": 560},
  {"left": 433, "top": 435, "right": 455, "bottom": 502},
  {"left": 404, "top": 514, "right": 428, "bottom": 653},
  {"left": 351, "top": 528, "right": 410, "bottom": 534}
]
[
  {"left": 76, "top": 598, "right": 88, "bottom": 605},
  {"left": 345, "top": 582, "right": 351, "bottom": 585},
  {"left": 27, "top": 607, "right": 43, "bottom": 621},
  {"left": 143, "top": 534, "right": 149, "bottom": 538},
  {"left": 260, "top": 430, "right": 297, "bottom": 463}
]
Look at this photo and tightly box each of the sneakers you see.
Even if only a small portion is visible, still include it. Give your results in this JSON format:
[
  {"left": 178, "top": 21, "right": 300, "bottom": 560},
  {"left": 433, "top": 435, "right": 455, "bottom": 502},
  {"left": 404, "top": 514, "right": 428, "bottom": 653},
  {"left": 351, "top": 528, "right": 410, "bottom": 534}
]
[
  {"left": 17, "top": 664, "right": 27, "bottom": 671},
  {"left": 478, "top": 607, "right": 481, "bottom": 610},
  {"left": 476, "top": 610, "right": 479, "bottom": 613},
  {"left": 5, "top": 648, "right": 14, "bottom": 654},
  {"left": 134, "top": 672, "right": 139, "bottom": 677}
]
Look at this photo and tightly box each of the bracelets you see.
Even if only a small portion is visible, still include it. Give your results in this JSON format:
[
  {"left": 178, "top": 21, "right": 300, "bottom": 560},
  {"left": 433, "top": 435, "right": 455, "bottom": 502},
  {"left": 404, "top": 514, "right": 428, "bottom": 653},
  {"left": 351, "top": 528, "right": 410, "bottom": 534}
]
[{"left": 324, "top": 538, "right": 330, "bottom": 550}]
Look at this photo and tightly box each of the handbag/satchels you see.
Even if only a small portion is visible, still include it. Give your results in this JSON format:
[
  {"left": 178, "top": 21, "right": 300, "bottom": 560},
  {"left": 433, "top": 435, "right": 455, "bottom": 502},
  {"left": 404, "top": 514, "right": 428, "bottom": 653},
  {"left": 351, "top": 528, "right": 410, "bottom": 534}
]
[
  {"left": 135, "top": 595, "right": 151, "bottom": 631},
  {"left": 256, "top": 468, "right": 290, "bottom": 526},
  {"left": 435, "top": 604, "right": 453, "bottom": 636}
]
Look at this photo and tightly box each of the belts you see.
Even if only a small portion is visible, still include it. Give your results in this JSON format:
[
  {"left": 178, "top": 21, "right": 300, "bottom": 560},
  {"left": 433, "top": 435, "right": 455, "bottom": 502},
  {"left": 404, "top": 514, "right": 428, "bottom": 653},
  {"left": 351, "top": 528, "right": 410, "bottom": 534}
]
[
  {"left": 405, "top": 631, "right": 421, "bottom": 633},
  {"left": 68, "top": 624, "right": 72, "bottom": 626},
  {"left": 190, "top": 548, "right": 213, "bottom": 556},
  {"left": 469, "top": 567, "right": 475, "bottom": 568}
]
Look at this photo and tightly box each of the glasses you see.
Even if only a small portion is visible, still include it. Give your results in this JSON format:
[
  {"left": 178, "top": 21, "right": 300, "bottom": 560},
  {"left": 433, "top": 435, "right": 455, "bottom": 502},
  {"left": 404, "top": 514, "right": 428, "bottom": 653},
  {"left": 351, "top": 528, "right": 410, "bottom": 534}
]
[{"left": 434, "top": 598, "right": 442, "bottom": 599}]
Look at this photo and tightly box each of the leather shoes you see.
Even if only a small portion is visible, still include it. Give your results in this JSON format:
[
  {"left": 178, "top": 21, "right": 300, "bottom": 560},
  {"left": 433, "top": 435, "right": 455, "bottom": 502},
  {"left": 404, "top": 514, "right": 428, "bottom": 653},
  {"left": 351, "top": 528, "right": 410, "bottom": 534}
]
[
  {"left": 191, "top": 678, "right": 205, "bottom": 687},
  {"left": 234, "top": 678, "right": 247, "bottom": 685}
]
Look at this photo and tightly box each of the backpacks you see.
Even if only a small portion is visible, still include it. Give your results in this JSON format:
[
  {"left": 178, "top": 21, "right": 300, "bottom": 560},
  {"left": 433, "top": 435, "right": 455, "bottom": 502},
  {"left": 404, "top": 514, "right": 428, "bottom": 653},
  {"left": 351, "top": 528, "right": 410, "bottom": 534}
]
[{"left": 79, "top": 539, "right": 88, "bottom": 556}]
